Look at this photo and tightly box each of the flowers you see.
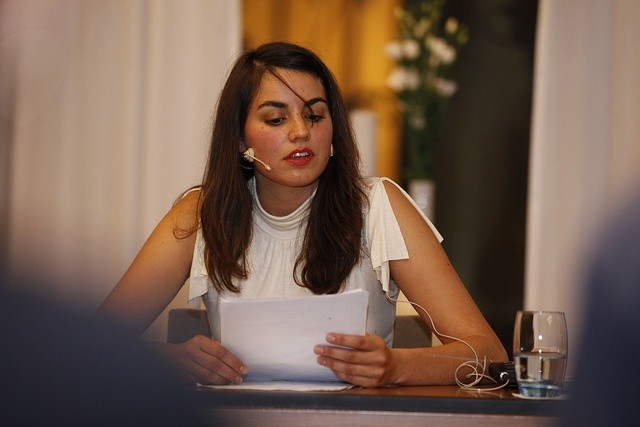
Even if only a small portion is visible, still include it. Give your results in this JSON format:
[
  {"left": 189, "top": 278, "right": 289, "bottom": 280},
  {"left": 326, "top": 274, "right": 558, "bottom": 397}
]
[{"left": 382, "top": 0, "right": 477, "bottom": 180}]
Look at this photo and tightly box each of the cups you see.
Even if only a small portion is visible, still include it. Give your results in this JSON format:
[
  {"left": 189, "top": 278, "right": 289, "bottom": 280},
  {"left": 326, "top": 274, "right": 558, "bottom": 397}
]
[{"left": 513, "top": 311, "right": 567, "bottom": 398}]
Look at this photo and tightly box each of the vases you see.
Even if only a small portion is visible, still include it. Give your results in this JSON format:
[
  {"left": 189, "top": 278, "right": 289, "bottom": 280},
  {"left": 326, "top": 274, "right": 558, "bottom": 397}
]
[{"left": 407, "top": 181, "right": 435, "bottom": 226}]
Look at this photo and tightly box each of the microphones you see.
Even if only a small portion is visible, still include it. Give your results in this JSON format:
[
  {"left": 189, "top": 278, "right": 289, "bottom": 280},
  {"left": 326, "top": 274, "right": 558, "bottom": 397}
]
[{"left": 243, "top": 148, "right": 272, "bottom": 172}]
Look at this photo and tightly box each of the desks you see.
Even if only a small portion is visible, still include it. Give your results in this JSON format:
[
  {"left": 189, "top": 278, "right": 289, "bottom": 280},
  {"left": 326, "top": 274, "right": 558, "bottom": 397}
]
[{"left": 201, "top": 379, "right": 572, "bottom": 427}]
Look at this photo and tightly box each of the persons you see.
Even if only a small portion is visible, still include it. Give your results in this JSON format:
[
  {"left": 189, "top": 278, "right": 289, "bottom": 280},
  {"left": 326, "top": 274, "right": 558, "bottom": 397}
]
[{"left": 91, "top": 42, "right": 509, "bottom": 388}]
